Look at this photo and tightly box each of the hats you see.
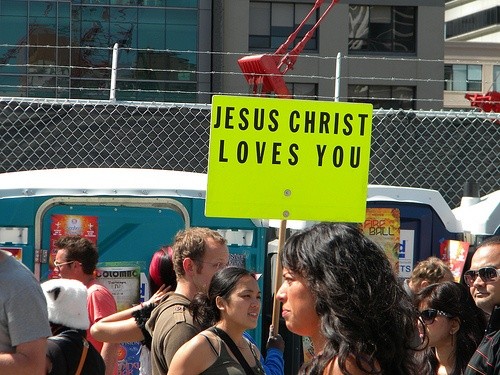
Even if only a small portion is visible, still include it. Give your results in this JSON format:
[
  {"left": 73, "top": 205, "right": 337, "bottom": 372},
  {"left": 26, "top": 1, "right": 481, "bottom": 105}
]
[{"left": 40, "top": 279, "right": 90, "bottom": 330}]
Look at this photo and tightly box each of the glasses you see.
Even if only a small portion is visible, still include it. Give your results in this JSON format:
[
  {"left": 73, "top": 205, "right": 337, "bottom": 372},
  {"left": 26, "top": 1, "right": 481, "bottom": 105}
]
[
  {"left": 54, "top": 261, "right": 82, "bottom": 270},
  {"left": 464, "top": 267, "right": 500, "bottom": 287},
  {"left": 419, "top": 309, "right": 455, "bottom": 325},
  {"left": 194, "top": 260, "right": 226, "bottom": 270}
]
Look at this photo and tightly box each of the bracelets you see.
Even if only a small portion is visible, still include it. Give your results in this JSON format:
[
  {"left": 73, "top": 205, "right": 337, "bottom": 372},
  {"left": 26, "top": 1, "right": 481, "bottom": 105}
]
[{"left": 139, "top": 301, "right": 143, "bottom": 308}]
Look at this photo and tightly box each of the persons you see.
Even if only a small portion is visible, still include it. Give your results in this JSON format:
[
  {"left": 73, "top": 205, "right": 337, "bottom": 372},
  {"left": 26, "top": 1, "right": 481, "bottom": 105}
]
[
  {"left": 464, "top": 235, "right": 500, "bottom": 375},
  {"left": 40, "top": 278, "right": 106, "bottom": 375},
  {"left": 90, "top": 227, "right": 285, "bottom": 375},
  {"left": 275, "top": 222, "right": 432, "bottom": 375},
  {"left": 401, "top": 256, "right": 490, "bottom": 375},
  {"left": 0, "top": 248, "right": 52, "bottom": 375},
  {"left": 54, "top": 235, "right": 119, "bottom": 375}
]
